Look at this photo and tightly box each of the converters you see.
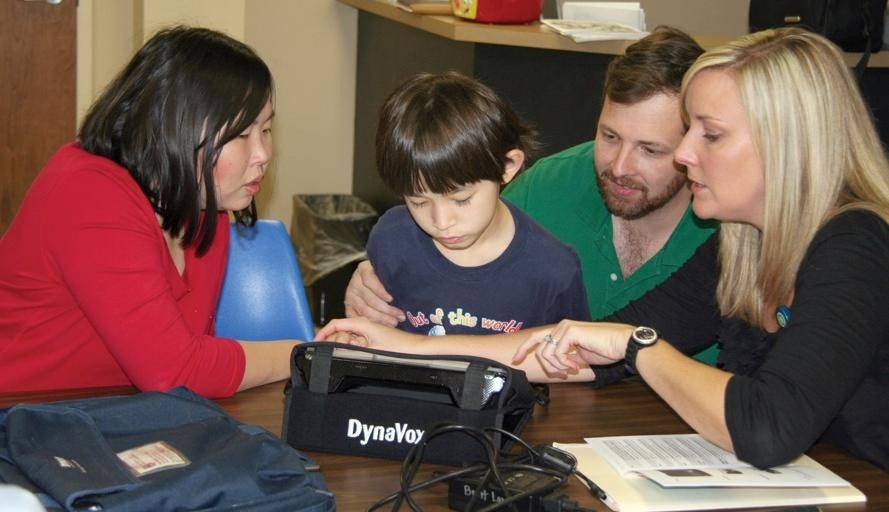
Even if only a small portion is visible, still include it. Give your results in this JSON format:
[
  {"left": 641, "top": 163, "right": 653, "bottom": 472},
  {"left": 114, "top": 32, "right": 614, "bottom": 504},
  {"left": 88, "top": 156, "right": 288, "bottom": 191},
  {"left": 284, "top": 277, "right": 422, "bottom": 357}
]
[{"left": 448, "top": 467, "right": 561, "bottom": 512}]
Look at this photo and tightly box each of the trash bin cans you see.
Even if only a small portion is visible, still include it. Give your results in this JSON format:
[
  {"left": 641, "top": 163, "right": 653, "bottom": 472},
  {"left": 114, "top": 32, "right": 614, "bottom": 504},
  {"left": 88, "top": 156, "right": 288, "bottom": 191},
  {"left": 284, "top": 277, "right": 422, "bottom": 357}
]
[{"left": 292, "top": 195, "right": 379, "bottom": 326}]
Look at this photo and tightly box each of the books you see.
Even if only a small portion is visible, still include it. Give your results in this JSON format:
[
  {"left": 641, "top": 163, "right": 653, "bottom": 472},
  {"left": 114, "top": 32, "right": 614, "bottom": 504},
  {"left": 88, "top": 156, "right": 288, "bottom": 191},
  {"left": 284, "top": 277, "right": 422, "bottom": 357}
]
[{"left": 551, "top": 439, "right": 868, "bottom": 511}]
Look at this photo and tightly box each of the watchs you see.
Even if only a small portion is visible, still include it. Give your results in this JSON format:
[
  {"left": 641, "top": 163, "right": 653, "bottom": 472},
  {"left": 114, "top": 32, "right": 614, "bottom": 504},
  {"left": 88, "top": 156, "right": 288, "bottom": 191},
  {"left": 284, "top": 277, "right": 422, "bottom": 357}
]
[{"left": 622, "top": 326, "right": 664, "bottom": 375}]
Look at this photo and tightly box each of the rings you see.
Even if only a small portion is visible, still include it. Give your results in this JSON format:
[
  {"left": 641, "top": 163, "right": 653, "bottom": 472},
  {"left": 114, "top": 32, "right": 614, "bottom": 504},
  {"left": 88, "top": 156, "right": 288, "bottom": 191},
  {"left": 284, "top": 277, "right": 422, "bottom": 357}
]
[
  {"left": 547, "top": 338, "right": 560, "bottom": 348},
  {"left": 539, "top": 331, "right": 552, "bottom": 344}
]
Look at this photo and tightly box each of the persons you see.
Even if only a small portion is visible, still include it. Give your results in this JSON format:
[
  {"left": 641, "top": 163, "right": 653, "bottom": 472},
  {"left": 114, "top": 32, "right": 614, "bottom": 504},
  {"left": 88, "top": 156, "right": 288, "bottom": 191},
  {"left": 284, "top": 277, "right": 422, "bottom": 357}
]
[
  {"left": 341, "top": 21, "right": 728, "bottom": 368},
  {"left": 359, "top": 71, "right": 585, "bottom": 350},
  {"left": 0, "top": 20, "right": 325, "bottom": 396},
  {"left": 313, "top": 26, "right": 889, "bottom": 473}
]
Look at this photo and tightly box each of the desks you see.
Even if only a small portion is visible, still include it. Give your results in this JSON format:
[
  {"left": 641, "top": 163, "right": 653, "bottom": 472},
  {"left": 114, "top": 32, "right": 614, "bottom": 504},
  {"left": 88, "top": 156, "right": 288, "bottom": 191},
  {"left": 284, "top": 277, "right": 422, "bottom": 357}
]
[{"left": 0, "top": 372, "right": 888, "bottom": 512}]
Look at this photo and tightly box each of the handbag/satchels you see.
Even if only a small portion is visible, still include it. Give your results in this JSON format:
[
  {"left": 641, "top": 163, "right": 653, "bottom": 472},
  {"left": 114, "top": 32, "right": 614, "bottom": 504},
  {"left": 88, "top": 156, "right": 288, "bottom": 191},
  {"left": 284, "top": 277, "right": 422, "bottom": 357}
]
[{"left": 2, "top": 386, "right": 339, "bottom": 511}]
